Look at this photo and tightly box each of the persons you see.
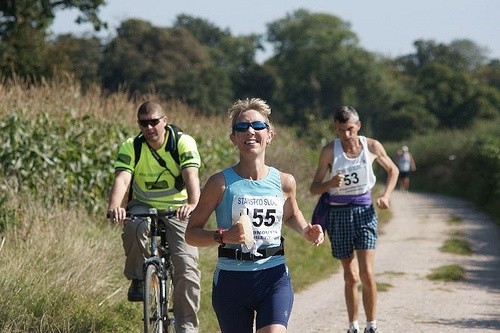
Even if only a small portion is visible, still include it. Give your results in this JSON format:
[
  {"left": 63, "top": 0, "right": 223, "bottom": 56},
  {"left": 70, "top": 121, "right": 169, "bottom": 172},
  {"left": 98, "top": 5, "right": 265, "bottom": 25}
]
[
  {"left": 397, "top": 146, "right": 417, "bottom": 192},
  {"left": 310, "top": 106, "right": 400, "bottom": 332},
  {"left": 185, "top": 99, "right": 323, "bottom": 333},
  {"left": 108, "top": 101, "right": 201, "bottom": 333}
]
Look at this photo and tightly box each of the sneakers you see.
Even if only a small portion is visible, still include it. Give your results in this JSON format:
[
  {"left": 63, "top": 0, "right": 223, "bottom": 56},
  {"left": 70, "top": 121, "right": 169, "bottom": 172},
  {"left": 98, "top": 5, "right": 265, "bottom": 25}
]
[{"left": 127, "top": 280, "right": 144, "bottom": 301}]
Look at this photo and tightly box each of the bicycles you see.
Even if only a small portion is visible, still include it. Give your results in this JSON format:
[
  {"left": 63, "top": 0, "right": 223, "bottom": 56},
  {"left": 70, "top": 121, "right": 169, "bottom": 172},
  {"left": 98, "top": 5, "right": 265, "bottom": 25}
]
[{"left": 105, "top": 205, "right": 195, "bottom": 333}]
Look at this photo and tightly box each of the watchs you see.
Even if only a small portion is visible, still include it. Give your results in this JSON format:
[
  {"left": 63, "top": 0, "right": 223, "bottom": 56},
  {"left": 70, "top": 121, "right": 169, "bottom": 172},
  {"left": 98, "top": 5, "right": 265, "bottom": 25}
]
[{"left": 214, "top": 227, "right": 227, "bottom": 245}]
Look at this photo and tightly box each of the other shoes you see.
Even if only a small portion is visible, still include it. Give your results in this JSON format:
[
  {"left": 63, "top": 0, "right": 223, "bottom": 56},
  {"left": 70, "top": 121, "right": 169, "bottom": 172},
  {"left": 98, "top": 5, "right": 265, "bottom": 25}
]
[
  {"left": 347, "top": 328, "right": 359, "bottom": 333},
  {"left": 364, "top": 327, "right": 378, "bottom": 333}
]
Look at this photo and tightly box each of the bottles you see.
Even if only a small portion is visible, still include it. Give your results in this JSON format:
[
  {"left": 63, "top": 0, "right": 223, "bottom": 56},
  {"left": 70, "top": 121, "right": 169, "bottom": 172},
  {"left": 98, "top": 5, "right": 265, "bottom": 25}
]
[{"left": 236, "top": 208, "right": 254, "bottom": 253}]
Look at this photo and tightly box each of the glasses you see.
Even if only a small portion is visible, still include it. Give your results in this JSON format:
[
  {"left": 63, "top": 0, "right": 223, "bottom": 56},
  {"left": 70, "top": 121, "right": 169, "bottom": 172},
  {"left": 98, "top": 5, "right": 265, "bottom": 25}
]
[
  {"left": 233, "top": 121, "right": 268, "bottom": 132},
  {"left": 139, "top": 115, "right": 165, "bottom": 128}
]
